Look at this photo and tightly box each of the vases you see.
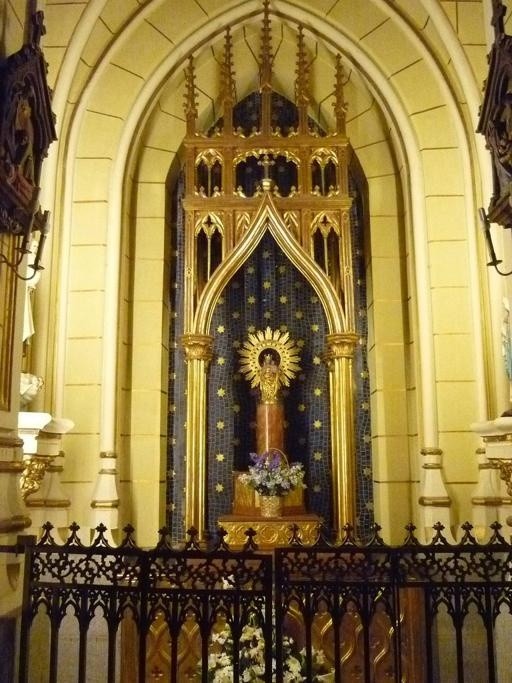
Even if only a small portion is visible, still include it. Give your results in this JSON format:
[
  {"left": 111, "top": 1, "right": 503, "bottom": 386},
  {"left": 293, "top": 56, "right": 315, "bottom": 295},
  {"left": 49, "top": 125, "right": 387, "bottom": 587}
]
[{"left": 259, "top": 496, "right": 284, "bottom": 518}]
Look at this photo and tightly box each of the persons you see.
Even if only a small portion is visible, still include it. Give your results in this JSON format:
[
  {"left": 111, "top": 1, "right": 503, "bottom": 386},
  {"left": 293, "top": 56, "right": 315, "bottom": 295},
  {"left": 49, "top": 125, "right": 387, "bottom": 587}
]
[{"left": 259, "top": 350, "right": 282, "bottom": 401}]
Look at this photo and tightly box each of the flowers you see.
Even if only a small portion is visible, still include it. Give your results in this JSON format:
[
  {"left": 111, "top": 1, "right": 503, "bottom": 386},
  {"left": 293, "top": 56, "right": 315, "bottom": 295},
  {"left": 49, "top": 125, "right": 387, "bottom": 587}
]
[
  {"left": 237, "top": 452, "right": 307, "bottom": 496},
  {"left": 197, "top": 574, "right": 335, "bottom": 683}
]
